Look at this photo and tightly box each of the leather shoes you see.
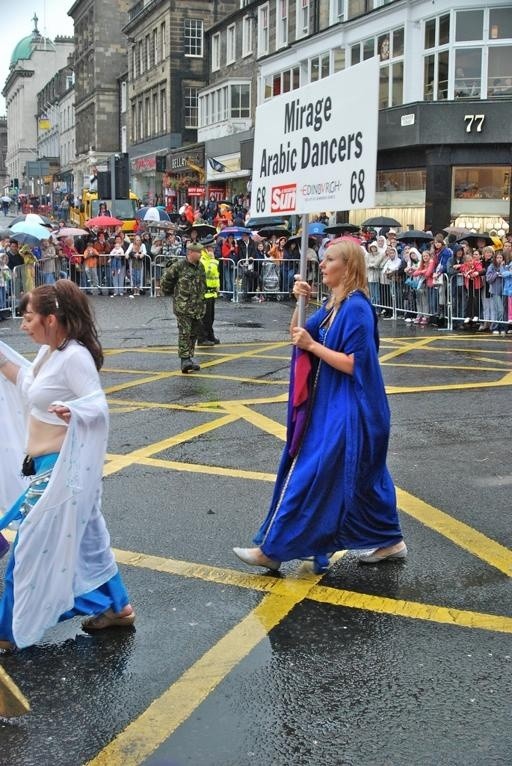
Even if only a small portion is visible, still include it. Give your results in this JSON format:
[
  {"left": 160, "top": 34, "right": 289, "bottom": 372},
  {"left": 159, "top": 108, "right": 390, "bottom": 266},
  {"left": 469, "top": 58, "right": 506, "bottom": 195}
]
[
  {"left": 360, "top": 544, "right": 408, "bottom": 565},
  {"left": 232, "top": 546, "right": 282, "bottom": 572}
]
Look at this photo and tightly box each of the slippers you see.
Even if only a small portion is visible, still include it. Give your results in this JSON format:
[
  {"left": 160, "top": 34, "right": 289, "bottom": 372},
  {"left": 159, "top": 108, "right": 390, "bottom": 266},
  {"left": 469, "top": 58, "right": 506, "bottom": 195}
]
[{"left": 81, "top": 611, "right": 135, "bottom": 630}]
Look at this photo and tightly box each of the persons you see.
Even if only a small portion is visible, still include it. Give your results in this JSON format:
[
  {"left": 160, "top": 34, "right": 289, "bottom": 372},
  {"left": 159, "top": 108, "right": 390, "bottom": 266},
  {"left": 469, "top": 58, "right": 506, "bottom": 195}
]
[
  {"left": 196, "top": 241, "right": 222, "bottom": 347},
  {"left": 0, "top": 277, "right": 138, "bottom": 653},
  {"left": 232, "top": 240, "right": 408, "bottom": 573},
  {"left": 159, "top": 241, "right": 209, "bottom": 375},
  {"left": 1, "top": 193, "right": 512, "bottom": 336},
  {"left": 422, "top": 68, "right": 512, "bottom": 100}
]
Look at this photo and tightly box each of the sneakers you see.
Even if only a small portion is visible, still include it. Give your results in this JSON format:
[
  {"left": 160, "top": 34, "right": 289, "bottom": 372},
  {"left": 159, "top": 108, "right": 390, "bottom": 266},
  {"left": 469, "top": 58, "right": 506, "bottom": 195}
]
[
  {"left": 180, "top": 358, "right": 201, "bottom": 372},
  {"left": 375, "top": 309, "right": 512, "bottom": 335},
  {"left": 217, "top": 293, "right": 260, "bottom": 302},
  {"left": 86, "top": 290, "right": 145, "bottom": 299}
]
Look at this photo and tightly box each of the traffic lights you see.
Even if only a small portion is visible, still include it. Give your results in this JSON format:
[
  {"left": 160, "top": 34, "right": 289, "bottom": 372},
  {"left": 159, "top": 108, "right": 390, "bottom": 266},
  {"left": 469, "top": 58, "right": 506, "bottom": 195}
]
[
  {"left": 11, "top": 180, "right": 13, "bottom": 188},
  {"left": 14, "top": 178, "right": 19, "bottom": 190}
]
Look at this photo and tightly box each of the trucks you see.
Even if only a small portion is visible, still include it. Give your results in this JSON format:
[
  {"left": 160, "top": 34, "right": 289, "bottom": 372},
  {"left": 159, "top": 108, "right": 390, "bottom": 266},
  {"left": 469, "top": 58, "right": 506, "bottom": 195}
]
[{"left": 68, "top": 188, "right": 142, "bottom": 243}]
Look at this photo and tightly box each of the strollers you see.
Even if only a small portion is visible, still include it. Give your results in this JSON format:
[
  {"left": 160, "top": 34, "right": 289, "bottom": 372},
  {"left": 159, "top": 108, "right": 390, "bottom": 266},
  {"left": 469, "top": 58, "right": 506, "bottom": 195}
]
[{"left": 262, "top": 261, "right": 285, "bottom": 302}]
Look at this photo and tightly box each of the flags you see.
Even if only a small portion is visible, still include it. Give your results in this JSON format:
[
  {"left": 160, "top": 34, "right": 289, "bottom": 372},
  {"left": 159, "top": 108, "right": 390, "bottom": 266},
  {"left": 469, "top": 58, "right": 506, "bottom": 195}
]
[{"left": 208, "top": 157, "right": 226, "bottom": 172}]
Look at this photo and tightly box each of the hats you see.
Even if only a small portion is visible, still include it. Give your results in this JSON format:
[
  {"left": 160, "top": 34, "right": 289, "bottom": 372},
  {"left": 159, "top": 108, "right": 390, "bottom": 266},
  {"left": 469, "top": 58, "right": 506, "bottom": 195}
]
[
  {"left": 186, "top": 242, "right": 204, "bottom": 251},
  {"left": 201, "top": 237, "right": 216, "bottom": 247}
]
